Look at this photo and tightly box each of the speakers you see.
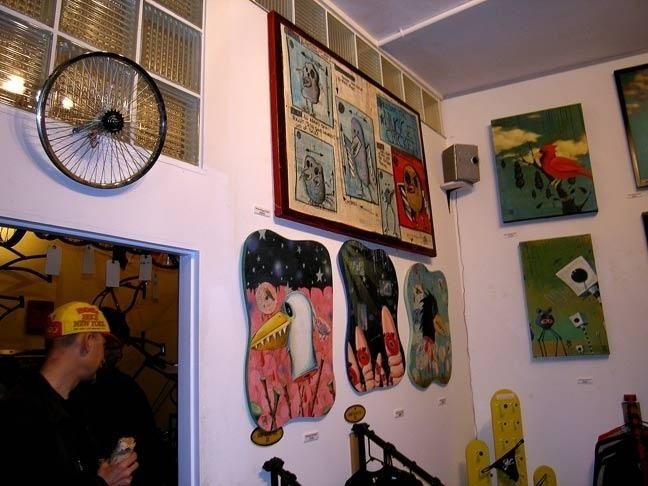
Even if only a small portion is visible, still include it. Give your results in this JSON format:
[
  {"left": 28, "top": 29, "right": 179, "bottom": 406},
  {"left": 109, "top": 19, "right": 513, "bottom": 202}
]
[{"left": 441, "top": 143, "right": 481, "bottom": 182}]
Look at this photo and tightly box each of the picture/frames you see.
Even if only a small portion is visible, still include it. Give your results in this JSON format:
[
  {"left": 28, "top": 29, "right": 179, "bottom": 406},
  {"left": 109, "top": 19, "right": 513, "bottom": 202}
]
[
  {"left": 268, "top": 9, "right": 436, "bottom": 257},
  {"left": 614, "top": 63, "right": 647, "bottom": 189}
]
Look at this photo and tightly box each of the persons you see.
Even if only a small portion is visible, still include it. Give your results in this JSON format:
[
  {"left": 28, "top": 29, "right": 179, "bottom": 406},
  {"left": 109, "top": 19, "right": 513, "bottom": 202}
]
[
  {"left": 100, "top": 305, "right": 157, "bottom": 438},
  {"left": 0, "top": 302, "right": 140, "bottom": 486}
]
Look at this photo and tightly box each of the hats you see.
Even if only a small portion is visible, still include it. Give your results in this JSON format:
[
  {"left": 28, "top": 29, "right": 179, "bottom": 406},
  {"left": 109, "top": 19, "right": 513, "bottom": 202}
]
[{"left": 45, "top": 301, "right": 120, "bottom": 347}]
